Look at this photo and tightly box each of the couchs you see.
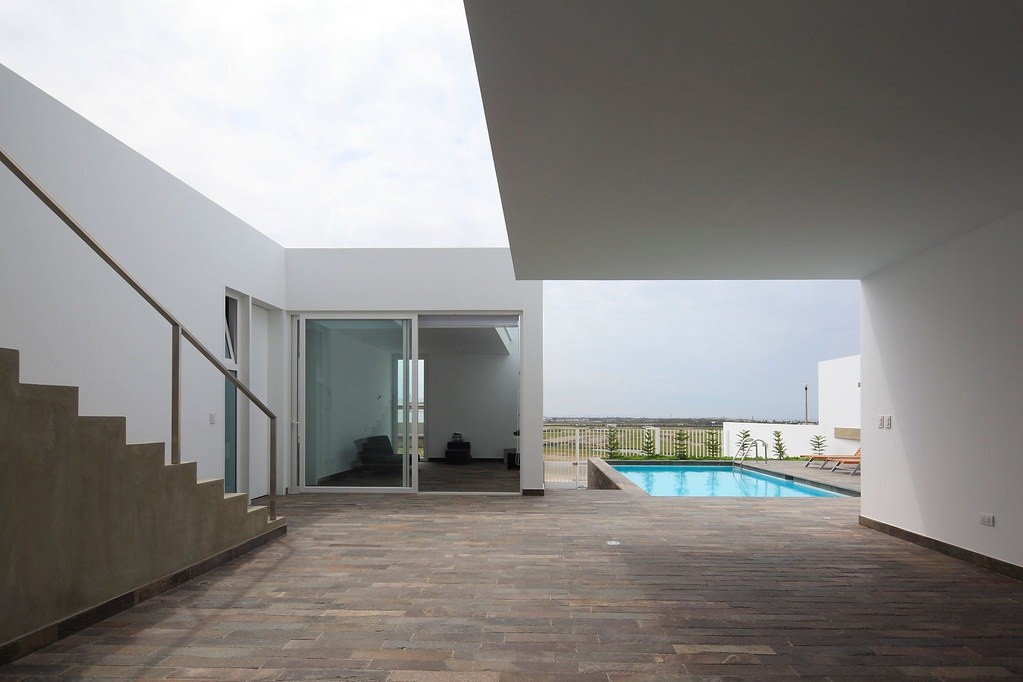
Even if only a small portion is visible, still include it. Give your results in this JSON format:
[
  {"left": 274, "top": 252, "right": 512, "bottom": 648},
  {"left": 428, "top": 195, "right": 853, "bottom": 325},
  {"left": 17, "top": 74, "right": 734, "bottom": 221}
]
[{"left": 354, "top": 435, "right": 420, "bottom": 475}]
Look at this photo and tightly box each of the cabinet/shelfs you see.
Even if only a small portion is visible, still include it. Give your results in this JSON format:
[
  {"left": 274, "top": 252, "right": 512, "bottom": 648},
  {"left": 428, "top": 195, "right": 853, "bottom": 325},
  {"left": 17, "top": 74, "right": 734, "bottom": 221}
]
[{"left": 503, "top": 449, "right": 518, "bottom": 470}]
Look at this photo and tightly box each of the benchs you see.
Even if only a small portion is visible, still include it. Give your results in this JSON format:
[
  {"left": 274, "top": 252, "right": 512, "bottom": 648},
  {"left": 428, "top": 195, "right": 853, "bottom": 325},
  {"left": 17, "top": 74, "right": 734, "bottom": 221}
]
[
  {"left": 799, "top": 446, "right": 862, "bottom": 470},
  {"left": 826, "top": 459, "right": 861, "bottom": 476}
]
